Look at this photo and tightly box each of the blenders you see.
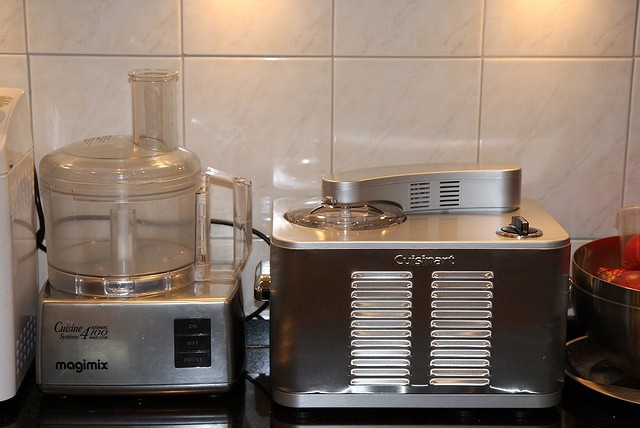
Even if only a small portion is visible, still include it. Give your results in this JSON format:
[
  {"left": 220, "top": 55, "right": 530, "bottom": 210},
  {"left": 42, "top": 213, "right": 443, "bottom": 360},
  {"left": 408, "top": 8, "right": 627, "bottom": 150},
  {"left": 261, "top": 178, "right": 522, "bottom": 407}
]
[{"left": 35, "top": 68, "right": 252, "bottom": 395}]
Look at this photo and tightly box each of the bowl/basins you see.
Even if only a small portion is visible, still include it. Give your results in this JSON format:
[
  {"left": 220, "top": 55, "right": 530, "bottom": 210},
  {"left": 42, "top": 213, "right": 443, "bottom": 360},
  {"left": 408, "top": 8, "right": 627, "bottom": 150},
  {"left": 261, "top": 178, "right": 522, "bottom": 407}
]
[{"left": 572, "top": 236, "right": 640, "bottom": 378}]
